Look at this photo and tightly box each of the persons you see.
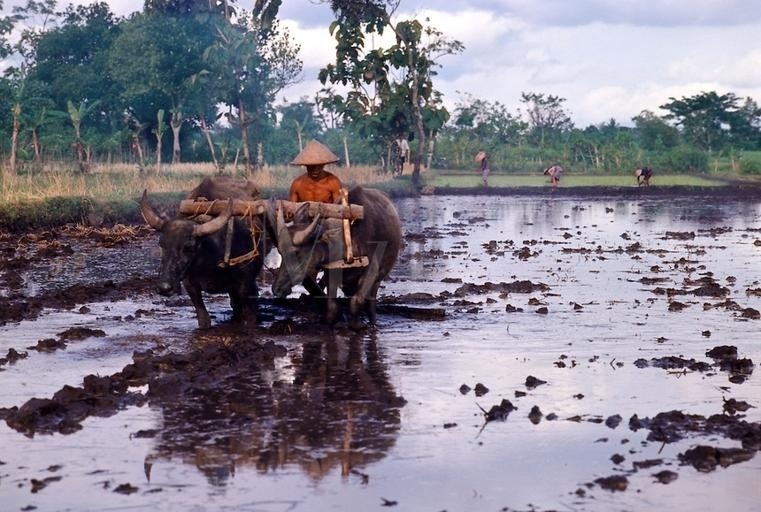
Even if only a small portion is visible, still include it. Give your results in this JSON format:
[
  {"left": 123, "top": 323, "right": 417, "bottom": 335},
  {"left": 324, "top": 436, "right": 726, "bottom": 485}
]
[
  {"left": 397, "top": 134, "right": 412, "bottom": 175},
  {"left": 634, "top": 167, "right": 654, "bottom": 188},
  {"left": 289, "top": 160, "right": 342, "bottom": 205},
  {"left": 542, "top": 165, "right": 563, "bottom": 187},
  {"left": 473, "top": 150, "right": 490, "bottom": 184}
]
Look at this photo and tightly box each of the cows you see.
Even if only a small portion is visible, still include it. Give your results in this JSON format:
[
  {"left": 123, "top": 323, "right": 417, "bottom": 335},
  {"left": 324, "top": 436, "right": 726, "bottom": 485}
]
[
  {"left": 271, "top": 185, "right": 402, "bottom": 331},
  {"left": 138, "top": 174, "right": 272, "bottom": 330}
]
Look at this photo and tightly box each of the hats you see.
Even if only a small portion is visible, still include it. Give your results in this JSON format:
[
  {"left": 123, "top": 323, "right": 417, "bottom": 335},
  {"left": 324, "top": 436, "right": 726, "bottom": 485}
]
[
  {"left": 543, "top": 167, "right": 548, "bottom": 175},
  {"left": 473, "top": 151, "right": 486, "bottom": 163},
  {"left": 290, "top": 137, "right": 340, "bottom": 165},
  {"left": 633, "top": 167, "right": 643, "bottom": 178}
]
